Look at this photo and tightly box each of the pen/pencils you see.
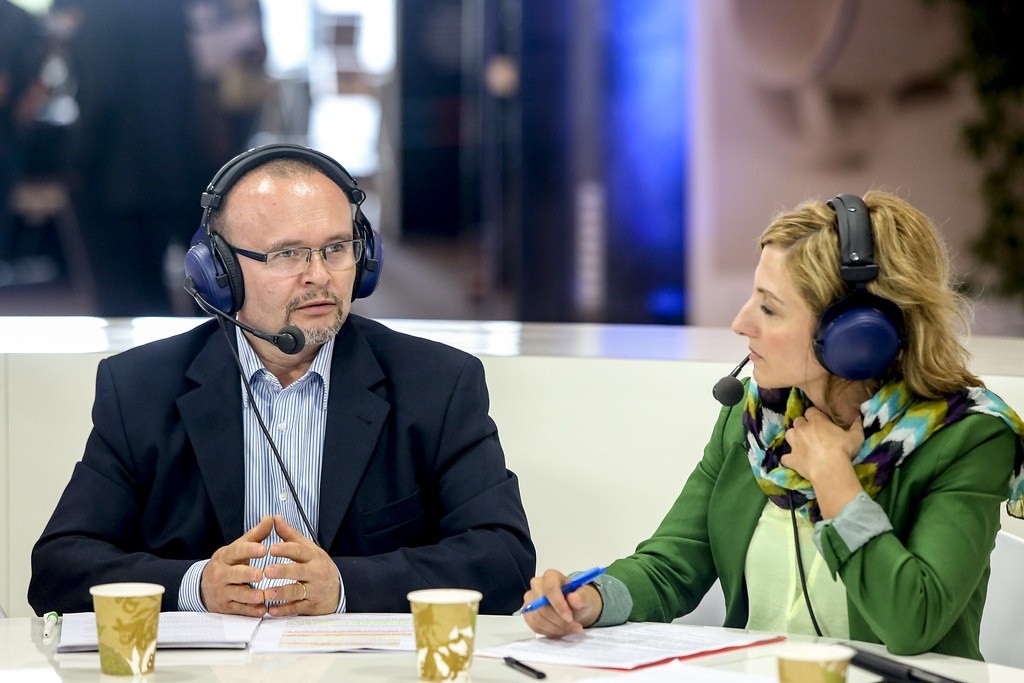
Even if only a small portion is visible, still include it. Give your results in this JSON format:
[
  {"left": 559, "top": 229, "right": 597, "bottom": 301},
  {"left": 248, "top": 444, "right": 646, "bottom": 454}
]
[
  {"left": 42, "top": 611, "right": 59, "bottom": 638},
  {"left": 511, "top": 566, "right": 608, "bottom": 620},
  {"left": 503, "top": 656, "right": 544, "bottom": 681}
]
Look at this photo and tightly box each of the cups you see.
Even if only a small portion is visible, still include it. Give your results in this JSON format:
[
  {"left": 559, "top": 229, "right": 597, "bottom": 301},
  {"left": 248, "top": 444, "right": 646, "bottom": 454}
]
[
  {"left": 776, "top": 642, "right": 857, "bottom": 683},
  {"left": 406, "top": 587, "right": 483, "bottom": 683},
  {"left": 89, "top": 582, "right": 166, "bottom": 677}
]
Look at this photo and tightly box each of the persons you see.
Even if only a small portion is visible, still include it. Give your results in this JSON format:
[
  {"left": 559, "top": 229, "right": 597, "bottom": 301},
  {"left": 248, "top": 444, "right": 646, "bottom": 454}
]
[
  {"left": 522, "top": 193, "right": 1023, "bottom": 666},
  {"left": 0, "top": 0, "right": 272, "bottom": 318},
  {"left": 26, "top": 143, "right": 536, "bottom": 619}
]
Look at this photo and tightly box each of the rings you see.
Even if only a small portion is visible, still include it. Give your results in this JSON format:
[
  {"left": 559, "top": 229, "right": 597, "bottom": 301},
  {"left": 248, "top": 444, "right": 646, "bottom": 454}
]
[{"left": 295, "top": 582, "right": 307, "bottom": 601}]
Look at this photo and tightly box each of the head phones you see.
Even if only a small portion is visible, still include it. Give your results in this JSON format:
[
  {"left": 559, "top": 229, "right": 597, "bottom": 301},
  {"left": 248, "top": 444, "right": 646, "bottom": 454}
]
[
  {"left": 808, "top": 193, "right": 909, "bottom": 380},
  {"left": 184, "top": 142, "right": 384, "bottom": 316}
]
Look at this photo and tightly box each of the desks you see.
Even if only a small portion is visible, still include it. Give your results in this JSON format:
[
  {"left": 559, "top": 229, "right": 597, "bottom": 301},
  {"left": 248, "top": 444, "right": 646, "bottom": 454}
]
[{"left": 0, "top": 614, "right": 1024, "bottom": 683}]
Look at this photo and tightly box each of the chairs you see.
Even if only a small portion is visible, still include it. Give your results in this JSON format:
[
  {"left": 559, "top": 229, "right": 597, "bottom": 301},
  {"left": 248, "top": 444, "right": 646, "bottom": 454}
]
[{"left": 979, "top": 531, "right": 1024, "bottom": 670}]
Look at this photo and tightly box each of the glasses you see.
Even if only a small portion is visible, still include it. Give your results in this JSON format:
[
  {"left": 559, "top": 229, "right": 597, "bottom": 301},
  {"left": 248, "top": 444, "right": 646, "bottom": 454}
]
[{"left": 229, "top": 234, "right": 364, "bottom": 277}]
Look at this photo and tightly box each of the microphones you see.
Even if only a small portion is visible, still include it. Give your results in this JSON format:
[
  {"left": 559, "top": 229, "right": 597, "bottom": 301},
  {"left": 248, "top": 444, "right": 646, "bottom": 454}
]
[
  {"left": 713, "top": 354, "right": 751, "bottom": 406},
  {"left": 183, "top": 277, "right": 306, "bottom": 356}
]
